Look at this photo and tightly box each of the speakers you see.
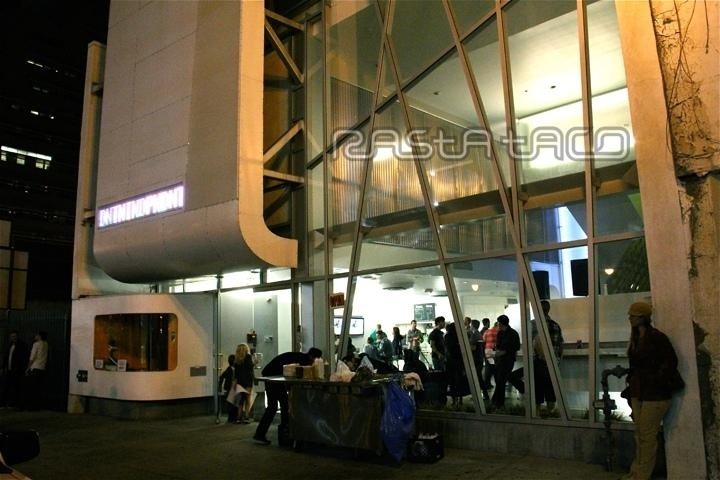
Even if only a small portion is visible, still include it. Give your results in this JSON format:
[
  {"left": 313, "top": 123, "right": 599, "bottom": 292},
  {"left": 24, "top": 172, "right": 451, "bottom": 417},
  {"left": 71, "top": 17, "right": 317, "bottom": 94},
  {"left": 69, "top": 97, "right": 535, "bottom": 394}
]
[
  {"left": 533, "top": 271, "right": 549, "bottom": 299},
  {"left": 570, "top": 258, "right": 601, "bottom": 295}
]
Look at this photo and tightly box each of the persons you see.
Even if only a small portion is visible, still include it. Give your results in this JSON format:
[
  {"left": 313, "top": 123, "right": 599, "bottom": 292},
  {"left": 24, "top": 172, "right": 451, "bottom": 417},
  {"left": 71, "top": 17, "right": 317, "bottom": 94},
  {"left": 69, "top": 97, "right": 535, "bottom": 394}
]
[
  {"left": 0, "top": 329, "right": 49, "bottom": 411},
  {"left": 618, "top": 302, "right": 686, "bottom": 480}
]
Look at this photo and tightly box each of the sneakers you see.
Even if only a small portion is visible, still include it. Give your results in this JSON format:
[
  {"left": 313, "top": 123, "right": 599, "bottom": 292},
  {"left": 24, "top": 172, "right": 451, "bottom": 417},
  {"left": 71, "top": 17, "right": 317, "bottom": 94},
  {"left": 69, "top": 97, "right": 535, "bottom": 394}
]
[
  {"left": 252, "top": 436, "right": 271, "bottom": 444},
  {"left": 235, "top": 418, "right": 241, "bottom": 423},
  {"left": 216, "top": 419, "right": 220, "bottom": 424},
  {"left": 243, "top": 418, "right": 249, "bottom": 423},
  {"left": 547, "top": 401, "right": 555, "bottom": 409}
]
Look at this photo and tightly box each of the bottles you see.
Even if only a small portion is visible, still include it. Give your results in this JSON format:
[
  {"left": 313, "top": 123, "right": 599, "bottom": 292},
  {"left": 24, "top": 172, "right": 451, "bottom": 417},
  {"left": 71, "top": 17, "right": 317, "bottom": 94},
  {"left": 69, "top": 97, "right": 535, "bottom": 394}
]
[{"left": 283, "top": 356, "right": 330, "bottom": 380}]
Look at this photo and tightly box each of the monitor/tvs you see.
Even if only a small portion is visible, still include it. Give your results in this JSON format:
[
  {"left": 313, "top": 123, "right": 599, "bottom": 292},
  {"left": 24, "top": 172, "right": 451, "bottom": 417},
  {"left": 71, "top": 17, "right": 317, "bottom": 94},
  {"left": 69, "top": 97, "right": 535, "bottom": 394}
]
[
  {"left": 349, "top": 317, "right": 364, "bottom": 336},
  {"left": 332, "top": 315, "right": 342, "bottom": 336}
]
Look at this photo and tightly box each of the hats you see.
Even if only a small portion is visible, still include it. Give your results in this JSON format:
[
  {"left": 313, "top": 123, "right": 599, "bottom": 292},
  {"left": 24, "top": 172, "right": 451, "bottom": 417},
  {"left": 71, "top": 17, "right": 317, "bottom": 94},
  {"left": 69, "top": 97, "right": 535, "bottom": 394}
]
[{"left": 628, "top": 303, "right": 651, "bottom": 317}]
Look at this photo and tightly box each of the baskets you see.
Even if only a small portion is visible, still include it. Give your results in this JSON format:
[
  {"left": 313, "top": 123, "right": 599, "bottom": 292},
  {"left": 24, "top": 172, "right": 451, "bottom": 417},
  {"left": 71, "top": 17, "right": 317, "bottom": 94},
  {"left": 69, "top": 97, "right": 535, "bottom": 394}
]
[{"left": 407, "top": 434, "right": 444, "bottom": 463}]
